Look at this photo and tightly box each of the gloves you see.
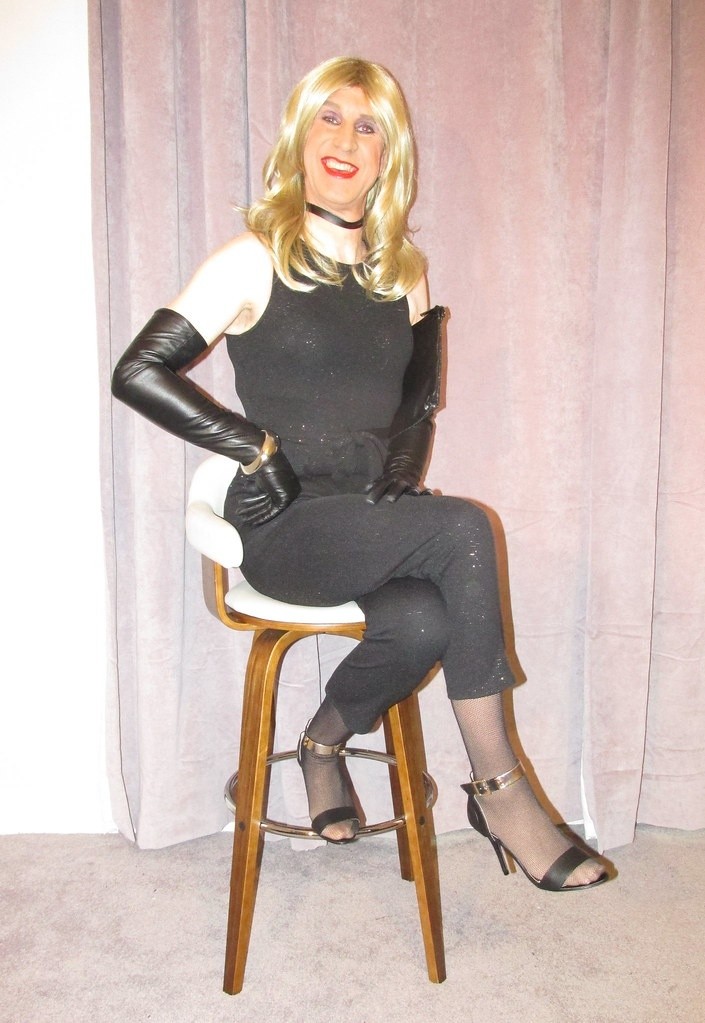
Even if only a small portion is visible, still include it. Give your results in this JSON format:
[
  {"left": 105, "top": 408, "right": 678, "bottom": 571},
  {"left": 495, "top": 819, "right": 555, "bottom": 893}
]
[
  {"left": 112, "top": 307, "right": 302, "bottom": 526},
  {"left": 364, "top": 305, "right": 445, "bottom": 505}
]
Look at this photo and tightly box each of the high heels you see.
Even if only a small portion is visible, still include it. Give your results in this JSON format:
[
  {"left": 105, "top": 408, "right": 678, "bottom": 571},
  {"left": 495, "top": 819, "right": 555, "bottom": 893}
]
[
  {"left": 297, "top": 718, "right": 360, "bottom": 846},
  {"left": 461, "top": 760, "right": 609, "bottom": 892}
]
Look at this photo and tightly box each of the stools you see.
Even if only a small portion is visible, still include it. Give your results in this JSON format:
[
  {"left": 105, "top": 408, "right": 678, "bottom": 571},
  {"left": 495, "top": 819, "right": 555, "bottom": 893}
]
[{"left": 187, "top": 453, "right": 447, "bottom": 996}]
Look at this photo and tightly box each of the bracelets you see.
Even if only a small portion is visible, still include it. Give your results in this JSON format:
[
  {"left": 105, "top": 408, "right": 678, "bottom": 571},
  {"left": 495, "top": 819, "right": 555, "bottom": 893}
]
[{"left": 240, "top": 429, "right": 281, "bottom": 475}]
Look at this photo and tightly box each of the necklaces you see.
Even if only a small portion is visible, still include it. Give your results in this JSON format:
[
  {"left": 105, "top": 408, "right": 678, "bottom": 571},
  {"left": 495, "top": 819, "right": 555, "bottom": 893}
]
[{"left": 304, "top": 201, "right": 368, "bottom": 229}]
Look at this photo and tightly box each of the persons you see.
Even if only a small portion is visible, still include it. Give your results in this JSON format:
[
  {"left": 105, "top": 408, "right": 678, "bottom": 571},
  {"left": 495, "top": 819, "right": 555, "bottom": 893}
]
[{"left": 112, "top": 57, "right": 609, "bottom": 893}]
